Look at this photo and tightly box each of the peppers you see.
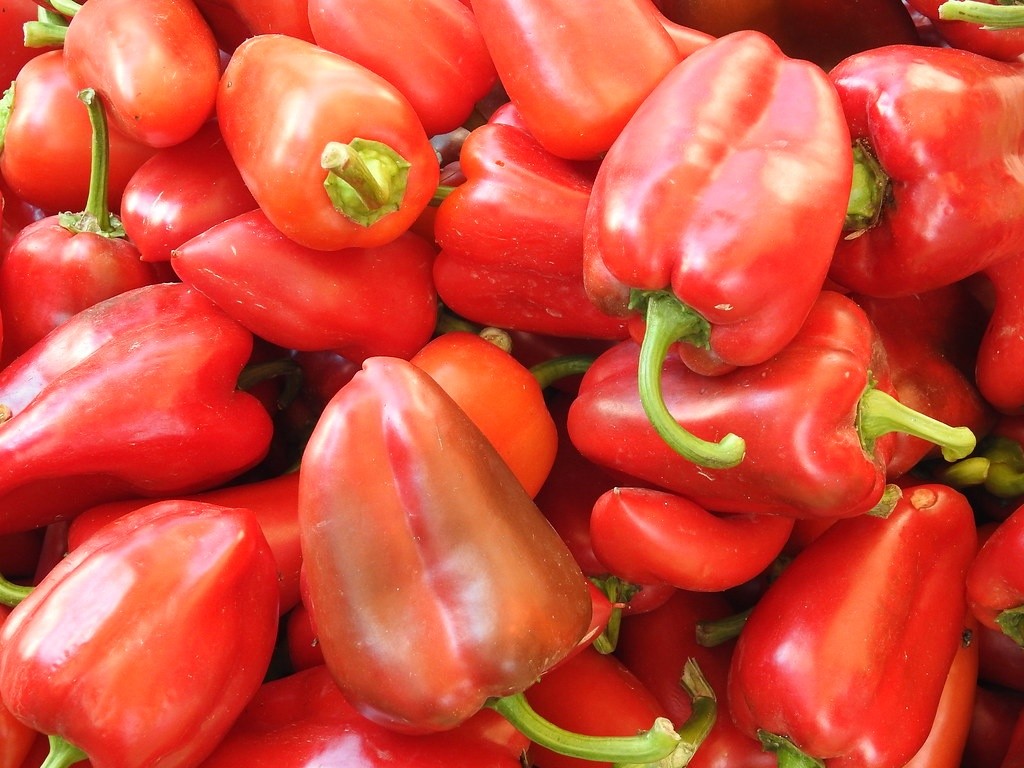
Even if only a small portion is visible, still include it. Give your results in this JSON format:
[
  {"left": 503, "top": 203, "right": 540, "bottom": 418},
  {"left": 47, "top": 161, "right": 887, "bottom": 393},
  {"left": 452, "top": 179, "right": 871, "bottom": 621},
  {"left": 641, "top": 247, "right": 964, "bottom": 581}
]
[{"left": 0, "top": 0, "right": 1024, "bottom": 768}]
